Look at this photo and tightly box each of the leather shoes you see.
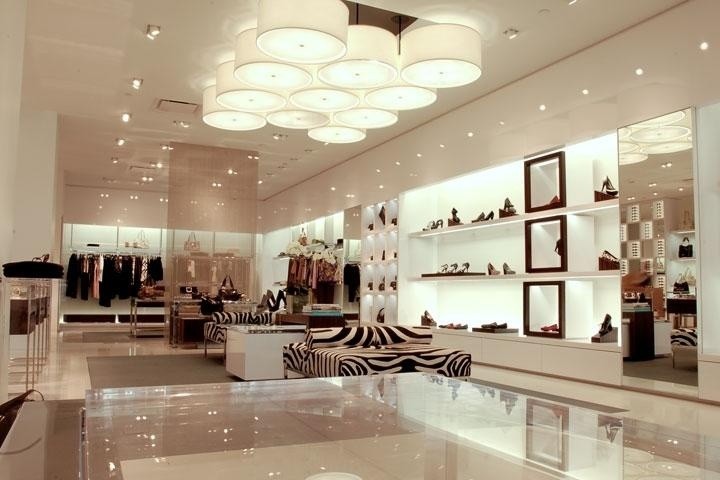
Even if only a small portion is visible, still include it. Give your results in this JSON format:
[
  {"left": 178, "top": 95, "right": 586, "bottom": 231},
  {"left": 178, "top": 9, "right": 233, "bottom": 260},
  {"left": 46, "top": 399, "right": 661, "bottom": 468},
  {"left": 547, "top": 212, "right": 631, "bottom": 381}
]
[
  {"left": 439, "top": 322, "right": 468, "bottom": 329},
  {"left": 482, "top": 322, "right": 508, "bottom": 329},
  {"left": 540, "top": 323, "right": 557, "bottom": 332}
]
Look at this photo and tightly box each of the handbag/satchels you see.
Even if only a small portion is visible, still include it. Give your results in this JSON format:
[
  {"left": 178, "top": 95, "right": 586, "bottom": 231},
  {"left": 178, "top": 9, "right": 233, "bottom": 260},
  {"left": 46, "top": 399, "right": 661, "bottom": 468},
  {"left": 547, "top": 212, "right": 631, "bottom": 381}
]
[
  {"left": 180, "top": 285, "right": 198, "bottom": 294},
  {"left": 379, "top": 276, "right": 385, "bottom": 290},
  {"left": 677, "top": 268, "right": 697, "bottom": 286},
  {"left": 672, "top": 281, "right": 690, "bottom": 295},
  {"left": 368, "top": 278, "right": 373, "bottom": 290},
  {"left": 392, "top": 215, "right": 398, "bottom": 224},
  {"left": 134, "top": 231, "right": 150, "bottom": 248},
  {"left": 368, "top": 219, "right": 374, "bottom": 231},
  {"left": 378, "top": 206, "right": 385, "bottom": 225},
  {"left": 217, "top": 275, "right": 240, "bottom": 301},
  {"left": 184, "top": 232, "right": 201, "bottom": 252},
  {"left": 678, "top": 236, "right": 693, "bottom": 258},
  {"left": 371, "top": 251, "right": 373, "bottom": 260},
  {"left": 200, "top": 295, "right": 224, "bottom": 315},
  {"left": 377, "top": 307, "right": 385, "bottom": 322},
  {"left": 390, "top": 275, "right": 397, "bottom": 290},
  {"left": 137, "top": 274, "right": 166, "bottom": 299},
  {"left": 680, "top": 210, "right": 693, "bottom": 230}
]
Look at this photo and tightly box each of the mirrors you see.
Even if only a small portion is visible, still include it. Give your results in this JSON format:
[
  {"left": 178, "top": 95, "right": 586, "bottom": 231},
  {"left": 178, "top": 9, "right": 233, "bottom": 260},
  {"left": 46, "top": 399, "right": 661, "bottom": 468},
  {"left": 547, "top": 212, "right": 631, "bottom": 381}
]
[{"left": 617, "top": 106, "right": 697, "bottom": 387}]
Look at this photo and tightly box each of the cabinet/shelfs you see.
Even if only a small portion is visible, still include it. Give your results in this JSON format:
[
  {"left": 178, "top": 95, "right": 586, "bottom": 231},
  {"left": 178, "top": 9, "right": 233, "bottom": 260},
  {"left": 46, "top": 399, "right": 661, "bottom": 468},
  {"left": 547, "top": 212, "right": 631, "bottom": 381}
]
[
  {"left": 169, "top": 300, "right": 212, "bottom": 348},
  {"left": 667, "top": 229, "right": 696, "bottom": 261},
  {"left": 525, "top": 399, "right": 599, "bottom": 473},
  {"left": 362, "top": 195, "right": 398, "bottom": 326},
  {"left": 410, "top": 198, "right": 620, "bottom": 282},
  {"left": 522, "top": 150, "right": 595, "bottom": 339},
  {"left": 433, "top": 329, "right": 622, "bottom": 389},
  {"left": 666, "top": 291, "right": 697, "bottom": 328}
]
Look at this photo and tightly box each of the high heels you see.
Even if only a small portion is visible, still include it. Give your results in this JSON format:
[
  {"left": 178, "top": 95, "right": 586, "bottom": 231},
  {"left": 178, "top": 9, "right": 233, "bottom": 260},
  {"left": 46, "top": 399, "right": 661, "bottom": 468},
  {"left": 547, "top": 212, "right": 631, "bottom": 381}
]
[
  {"left": 555, "top": 239, "right": 562, "bottom": 255},
  {"left": 471, "top": 210, "right": 495, "bottom": 223},
  {"left": 503, "top": 198, "right": 514, "bottom": 211},
  {"left": 603, "top": 250, "right": 621, "bottom": 263},
  {"left": 256, "top": 290, "right": 287, "bottom": 313},
  {"left": 422, "top": 219, "right": 444, "bottom": 232},
  {"left": 599, "top": 313, "right": 613, "bottom": 335},
  {"left": 452, "top": 208, "right": 460, "bottom": 223},
  {"left": 487, "top": 263, "right": 501, "bottom": 275},
  {"left": 440, "top": 263, "right": 470, "bottom": 274},
  {"left": 502, "top": 262, "right": 515, "bottom": 275},
  {"left": 601, "top": 175, "right": 618, "bottom": 196},
  {"left": 424, "top": 310, "right": 434, "bottom": 323},
  {"left": 546, "top": 195, "right": 558, "bottom": 206}
]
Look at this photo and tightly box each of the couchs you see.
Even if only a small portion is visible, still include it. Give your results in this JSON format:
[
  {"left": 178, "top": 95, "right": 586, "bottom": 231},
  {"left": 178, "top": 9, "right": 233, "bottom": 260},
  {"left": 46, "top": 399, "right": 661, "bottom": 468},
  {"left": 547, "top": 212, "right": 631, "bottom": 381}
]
[
  {"left": 282, "top": 326, "right": 471, "bottom": 380},
  {"left": 672, "top": 329, "right": 697, "bottom": 369},
  {"left": 203, "top": 312, "right": 281, "bottom": 361}
]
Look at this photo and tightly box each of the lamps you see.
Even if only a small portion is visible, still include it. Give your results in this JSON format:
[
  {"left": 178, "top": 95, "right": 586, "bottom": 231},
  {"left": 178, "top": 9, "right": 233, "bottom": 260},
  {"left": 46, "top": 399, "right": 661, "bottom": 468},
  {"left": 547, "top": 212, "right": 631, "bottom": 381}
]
[
  {"left": 619, "top": 109, "right": 693, "bottom": 165},
  {"left": 146, "top": 25, "right": 160, "bottom": 40}
]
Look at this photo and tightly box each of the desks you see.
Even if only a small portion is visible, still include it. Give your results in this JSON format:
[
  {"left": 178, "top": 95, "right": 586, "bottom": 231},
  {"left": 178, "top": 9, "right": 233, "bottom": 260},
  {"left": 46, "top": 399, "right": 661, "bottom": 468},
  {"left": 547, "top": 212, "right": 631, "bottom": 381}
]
[
  {"left": 226, "top": 326, "right": 307, "bottom": 380},
  {"left": 130, "top": 297, "right": 165, "bottom": 338},
  {"left": 279, "top": 313, "right": 345, "bottom": 327},
  {"left": 621, "top": 311, "right": 672, "bottom": 362}
]
